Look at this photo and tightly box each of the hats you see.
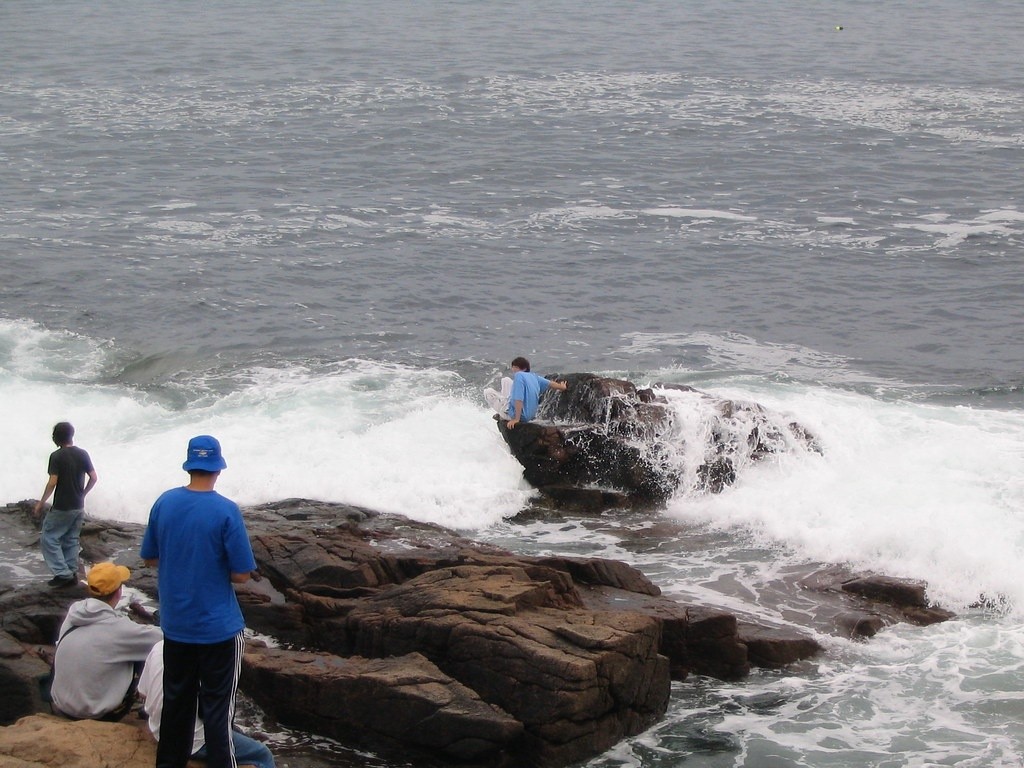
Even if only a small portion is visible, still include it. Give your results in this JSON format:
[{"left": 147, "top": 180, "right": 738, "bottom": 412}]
[
  {"left": 182, "top": 435, "right": 227, "bottom": 471},
  {"left": 85, "top": 562, "right": 131, "bottom": 596}
]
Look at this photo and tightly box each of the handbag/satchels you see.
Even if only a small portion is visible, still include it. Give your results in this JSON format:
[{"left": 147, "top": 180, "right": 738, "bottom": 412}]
[{"left": 39, "top": 673, "right": 55, "bottom": 701}]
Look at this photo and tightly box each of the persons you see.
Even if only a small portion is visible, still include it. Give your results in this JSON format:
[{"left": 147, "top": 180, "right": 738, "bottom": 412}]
[
  {"left": 140, "top": 434, "right": 259, "bottom": 768},
  {"left": 135, "top": 636, "right": 276, "bottom": 768},
  {"left": 35, "top": 421, "right": 96, "bottom": 587},
  {"left": 49, "top": 561, "right": 164, "bottom": 724},
  {"left": 484, "top": 356, "right": 568, "bottom": 430}
]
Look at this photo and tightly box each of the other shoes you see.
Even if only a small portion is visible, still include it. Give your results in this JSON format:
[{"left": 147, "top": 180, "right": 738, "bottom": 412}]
[
  {"left": 48, "top": 573, "right": 76, "bottom": 586},
  {"left": 66, "top": 572, "right": 79, "bottom": 586}
]
